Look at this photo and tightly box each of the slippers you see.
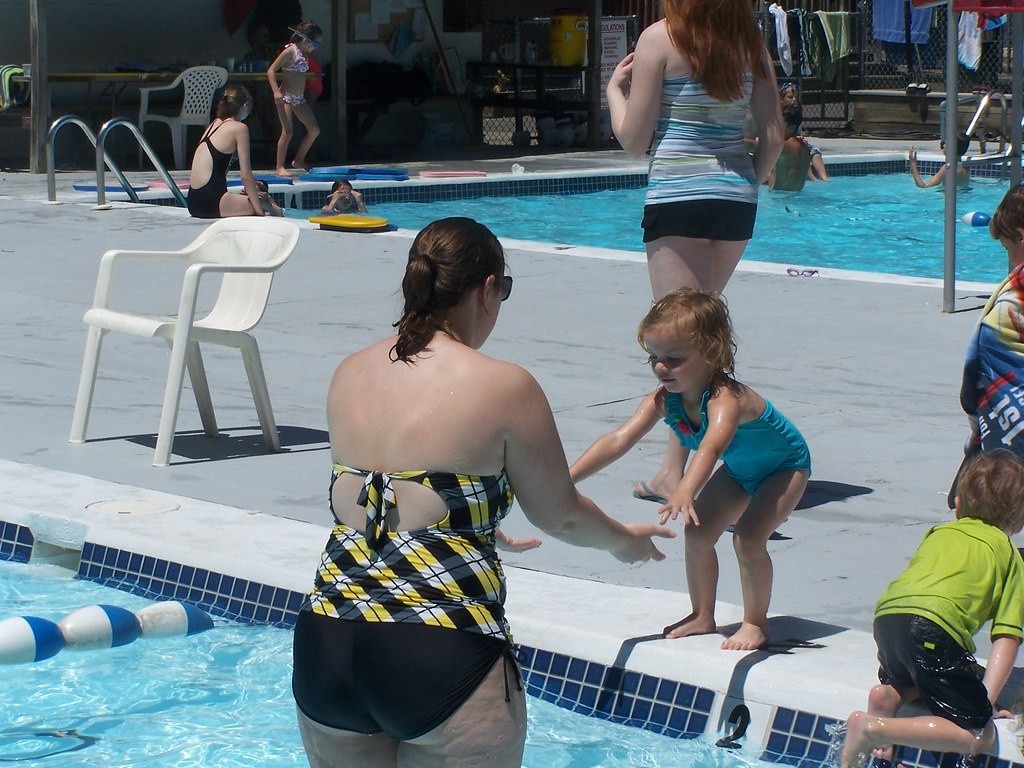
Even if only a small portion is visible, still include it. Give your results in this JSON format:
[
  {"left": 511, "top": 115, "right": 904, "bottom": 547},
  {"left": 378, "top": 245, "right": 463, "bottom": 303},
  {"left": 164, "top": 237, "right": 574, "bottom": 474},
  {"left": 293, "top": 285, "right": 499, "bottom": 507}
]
[
  {"left": 723, "top": 524, "right": 734, "bottom": 531},
  {"left": 634, "top": 479, "right": 667, "bottom": 505}
]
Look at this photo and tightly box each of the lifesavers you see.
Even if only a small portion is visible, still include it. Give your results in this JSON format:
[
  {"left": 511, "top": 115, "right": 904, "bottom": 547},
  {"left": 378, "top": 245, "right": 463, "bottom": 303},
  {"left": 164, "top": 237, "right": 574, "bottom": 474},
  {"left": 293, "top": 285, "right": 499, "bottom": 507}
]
[{"left": 0, "top": 64, "right": 31, "bottom": 110}]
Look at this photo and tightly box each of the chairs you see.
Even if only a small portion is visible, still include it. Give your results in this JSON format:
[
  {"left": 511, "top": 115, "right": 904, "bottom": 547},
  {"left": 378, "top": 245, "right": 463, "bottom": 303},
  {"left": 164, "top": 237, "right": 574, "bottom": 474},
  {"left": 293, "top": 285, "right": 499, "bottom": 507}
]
[
  {"left": 138, "top": 64, "right": 228, "bottom": 171},
  {"left": 67, "top": 215, "right": 300, "bottom": 467}
]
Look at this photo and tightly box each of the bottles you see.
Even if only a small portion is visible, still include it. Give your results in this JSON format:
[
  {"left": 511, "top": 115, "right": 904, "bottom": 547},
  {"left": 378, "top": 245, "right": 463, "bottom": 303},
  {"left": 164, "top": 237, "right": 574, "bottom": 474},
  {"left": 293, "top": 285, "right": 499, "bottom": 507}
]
[{"left": 238, "top": 61, "right": 253, "bottom": 72}]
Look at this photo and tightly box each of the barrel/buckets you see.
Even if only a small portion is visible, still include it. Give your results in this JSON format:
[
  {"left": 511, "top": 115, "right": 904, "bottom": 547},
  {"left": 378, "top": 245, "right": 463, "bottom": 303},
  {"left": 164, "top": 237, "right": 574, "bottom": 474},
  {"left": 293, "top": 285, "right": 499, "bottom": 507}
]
[{"left": 547, "top": 10, "right": 586, "bottom": 66}]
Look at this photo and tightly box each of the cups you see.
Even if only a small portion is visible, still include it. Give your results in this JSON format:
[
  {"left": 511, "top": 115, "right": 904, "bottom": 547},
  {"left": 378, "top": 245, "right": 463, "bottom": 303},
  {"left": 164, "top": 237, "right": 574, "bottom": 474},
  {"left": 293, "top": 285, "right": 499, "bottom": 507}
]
[
  {"left": 21, "top": 63, "right": 32, "bottom": 77},
  {"left": 226, "top": 57, "right": 235, "bottom": 72}
]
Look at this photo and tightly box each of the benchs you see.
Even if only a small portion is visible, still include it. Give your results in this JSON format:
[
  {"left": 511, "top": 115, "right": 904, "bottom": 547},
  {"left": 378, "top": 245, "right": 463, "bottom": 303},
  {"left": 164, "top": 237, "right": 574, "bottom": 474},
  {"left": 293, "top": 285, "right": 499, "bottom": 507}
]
[{"left": 848, "top": 88, "right": 1024, "bottom": 144}]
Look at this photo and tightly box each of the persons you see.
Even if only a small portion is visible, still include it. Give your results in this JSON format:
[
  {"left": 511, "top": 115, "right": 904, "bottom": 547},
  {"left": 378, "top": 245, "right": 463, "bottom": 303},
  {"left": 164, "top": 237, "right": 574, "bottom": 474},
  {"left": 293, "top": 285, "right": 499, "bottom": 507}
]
[
  {"left": 266, "top": 20, "right": 324, "bottom": 177},
  {"left": 187, "top": 85, "right": 282, "bottom": 219},
  {"left": 959, "top": 183, "right": 1024, "bottom": 468},
  {"left": 292, "top": 217, "right": 676, "bottom": 767},
  {"left": 566, "top": 286, "right": 811, "bottom": 650},
  {"left": 607, "top": 0, "right": 786, "bottom": 301},
  {"left": 322, "top": 180, "right": 368, "bottom": 213},
  {"left": 240, "top": 179, "right": 273, "bottom": 204},
  {"left": 743, "top": 83, "right": 827, "bottom": 191},
  {"left": 909, "top": 131, "right": 969, "bottom": 188},
  {"left": 841, "top": 445, "right": 1024, "bottom": 768}
]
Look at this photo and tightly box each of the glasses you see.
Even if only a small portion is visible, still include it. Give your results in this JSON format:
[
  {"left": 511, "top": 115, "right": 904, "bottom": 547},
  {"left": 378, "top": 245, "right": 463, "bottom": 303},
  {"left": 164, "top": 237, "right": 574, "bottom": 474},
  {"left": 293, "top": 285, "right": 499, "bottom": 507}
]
[
  {"left": 491, "top": 276, "right": 513, "bottom": 302},
  {"left": 341, "top": 191, "right": 353, "bottom": 197},
  {"left": 312, "top": 41, "right": 321, "bottom": 48},
  {"left": 787, "top": 268, "right": 820, "bottom": 277}
]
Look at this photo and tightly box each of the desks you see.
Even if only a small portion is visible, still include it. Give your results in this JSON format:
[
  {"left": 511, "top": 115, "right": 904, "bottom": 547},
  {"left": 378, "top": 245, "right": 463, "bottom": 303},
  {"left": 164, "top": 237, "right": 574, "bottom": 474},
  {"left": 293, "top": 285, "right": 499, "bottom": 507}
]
[{"left": 0, "top": 72, "right": 326, "bottom": 172}]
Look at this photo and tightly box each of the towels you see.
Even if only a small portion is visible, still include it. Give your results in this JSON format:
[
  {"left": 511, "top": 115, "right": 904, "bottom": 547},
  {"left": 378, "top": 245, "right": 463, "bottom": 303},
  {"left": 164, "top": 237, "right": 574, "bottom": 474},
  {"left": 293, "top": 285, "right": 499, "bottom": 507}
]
[
  {"left": 768, "top": 2, "right": 793, "bottom": 77},
  {"left": 0, "top": 63, "right": 32, "bottom": 111},
  {"left": 809, "top": 10, "right": 854, "bottom": 104},
  {"left": 956, "top": 10, "right": 983, "bottom": 72},
  {"left": 871, "top": 0, "right": 943, "bottom": 68}
]
